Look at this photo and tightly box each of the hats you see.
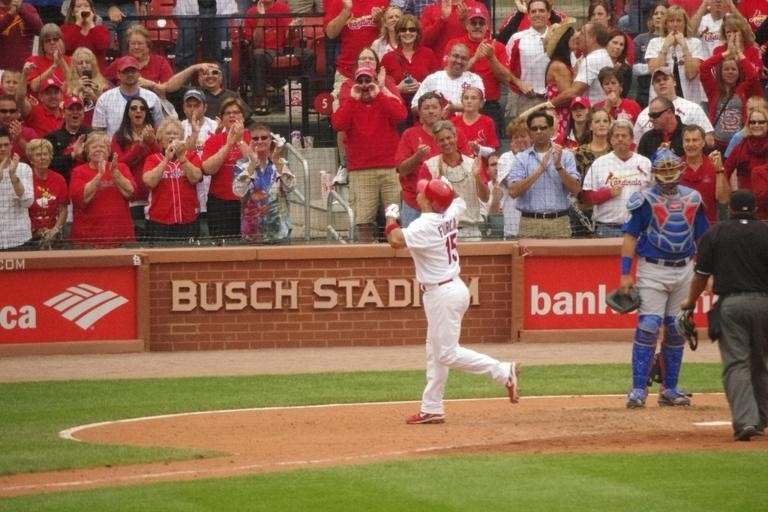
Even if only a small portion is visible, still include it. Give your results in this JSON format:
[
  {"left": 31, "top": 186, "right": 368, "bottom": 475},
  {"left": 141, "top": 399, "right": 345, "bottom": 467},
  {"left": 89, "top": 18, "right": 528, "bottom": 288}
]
[
  {"left": 118, "top": 56, "right": 141, "bottom": 71},
  {"left": 184, "top": 89, "right": 207, "bottom": 103},
  {"left": 730, "top": 190, "right": 754, "bottom": 212},
  {"left": 467, "top": 3, "right": 489, "bottom": 20},
  {"left": 38, "top": 77, "right": 63, "bottom": 93},
  {"left": 63, "top": 95, "right": 84, "bottom": 108}
]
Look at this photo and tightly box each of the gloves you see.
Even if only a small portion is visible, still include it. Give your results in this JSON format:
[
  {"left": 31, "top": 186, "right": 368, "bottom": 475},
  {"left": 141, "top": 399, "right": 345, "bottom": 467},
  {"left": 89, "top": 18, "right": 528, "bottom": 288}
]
[{"left": 385, "top": 204, "right": 399, "bottom": 218}]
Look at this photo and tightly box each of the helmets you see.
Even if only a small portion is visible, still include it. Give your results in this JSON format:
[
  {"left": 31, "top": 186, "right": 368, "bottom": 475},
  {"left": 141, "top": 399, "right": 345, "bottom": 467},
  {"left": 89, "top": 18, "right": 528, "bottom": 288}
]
[
  {"left": 651, "top": 146, "right": 686, "bottom": 191},
  {"left": 417, "top": 179, "right": 453, "bottom": 213}
]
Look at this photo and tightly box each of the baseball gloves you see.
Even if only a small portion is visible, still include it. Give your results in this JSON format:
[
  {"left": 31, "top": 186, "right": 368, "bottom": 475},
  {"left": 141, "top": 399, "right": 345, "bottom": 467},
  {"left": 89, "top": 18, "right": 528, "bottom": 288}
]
[
  {"left": 606, "top": 286, "right": 642, "bottom": 311},
  {"left": 676, "top": 308, "right": 698, "bottom": 352}
]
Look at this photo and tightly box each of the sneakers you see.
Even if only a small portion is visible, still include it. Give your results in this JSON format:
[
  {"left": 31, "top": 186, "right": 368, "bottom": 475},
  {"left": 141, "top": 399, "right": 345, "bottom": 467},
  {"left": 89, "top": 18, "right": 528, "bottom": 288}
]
[
  {"left": 734, "top": 425, "right": 754, "bottom": 440},
  {"left": 627, "top": 388, "right": 646, "bottom": 406},
  {"left": 407, "top": 412, "right": 444, "bottom": 424},
  {"left": 658, "top": 386, "right": 689, "bottom": 405},
  {"left": 506, "top": 363, "right": 520, "bottom": 402}
]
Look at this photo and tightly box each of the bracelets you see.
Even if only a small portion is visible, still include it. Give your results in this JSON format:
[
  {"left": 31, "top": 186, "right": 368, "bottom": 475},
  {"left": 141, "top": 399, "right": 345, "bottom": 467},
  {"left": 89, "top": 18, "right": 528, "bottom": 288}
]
[
  {"left": 382, "top": 223, "right": 401, "bottom": 238},
  {"left": 623, "top": 255, "right": 631, "bottom": 278}
]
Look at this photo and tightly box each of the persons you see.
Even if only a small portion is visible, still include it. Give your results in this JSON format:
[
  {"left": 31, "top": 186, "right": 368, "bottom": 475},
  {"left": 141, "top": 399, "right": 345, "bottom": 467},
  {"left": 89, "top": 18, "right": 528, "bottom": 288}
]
[
  {"left": 680, "top": 188, "right": 768, "bottom": 440},
  {"left": 382, "top": 174, "right": 523, "bottom": 426},
  {"left": 0, "top": 1, "right": 768, "bottom": 246},
  {"left": 619, "top": 145, "right": 708, "bottom": 410}
]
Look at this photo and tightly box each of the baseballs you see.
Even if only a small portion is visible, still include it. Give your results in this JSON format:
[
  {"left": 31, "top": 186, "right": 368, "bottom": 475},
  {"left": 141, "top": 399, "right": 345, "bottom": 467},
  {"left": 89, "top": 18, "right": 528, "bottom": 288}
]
[{"left": 158, "top": 17, "right": 169, "bottom": 26}]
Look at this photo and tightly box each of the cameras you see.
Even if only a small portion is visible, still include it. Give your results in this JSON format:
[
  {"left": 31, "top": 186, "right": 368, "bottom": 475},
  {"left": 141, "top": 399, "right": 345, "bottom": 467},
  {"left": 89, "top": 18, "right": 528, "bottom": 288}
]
[
  {"left": 208, "top": 69, "right": 220, "bottom": 78},
  {"left": 81, "top": 11, "right": 90, "bottom": 17}
]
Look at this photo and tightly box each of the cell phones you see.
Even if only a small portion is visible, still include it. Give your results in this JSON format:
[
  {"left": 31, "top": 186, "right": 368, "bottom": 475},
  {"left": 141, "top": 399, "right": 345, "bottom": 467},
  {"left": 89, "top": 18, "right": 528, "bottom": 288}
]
[{"left": 83, "top": 70, "right": 92, "bottom": 86}]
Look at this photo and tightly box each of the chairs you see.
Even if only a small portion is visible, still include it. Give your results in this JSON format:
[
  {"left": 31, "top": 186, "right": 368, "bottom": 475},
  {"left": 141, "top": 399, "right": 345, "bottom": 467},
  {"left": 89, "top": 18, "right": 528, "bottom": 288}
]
[{"left": 139, "top": 0, "right": 329, "bottom": 95}]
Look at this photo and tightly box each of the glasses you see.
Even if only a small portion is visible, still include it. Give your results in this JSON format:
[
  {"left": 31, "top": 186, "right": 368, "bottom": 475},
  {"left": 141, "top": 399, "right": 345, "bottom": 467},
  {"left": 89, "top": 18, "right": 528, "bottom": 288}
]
[
  {"left": 252, "top": 135, "right": 268, "bottom": 142},
  {"left": 131, "top": 104, "right": 146, "bottom": 112},
  {"left": 1, "top": 108, "right": 17, "bottom": 114},
  {"left": 44, "top": 36, "right": 59, "bottom": 43}
]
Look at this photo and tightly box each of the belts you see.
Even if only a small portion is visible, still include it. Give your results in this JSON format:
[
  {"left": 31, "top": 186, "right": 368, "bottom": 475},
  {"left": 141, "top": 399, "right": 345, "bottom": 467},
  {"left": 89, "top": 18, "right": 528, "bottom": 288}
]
[
  {"left": 645, "top": 256, "right": 685, "bottom": 266},
  {"left": 522, "top": 211, "right": 568, "bottom": 218},
  {"left": 439, "top": 279, "right": 453, "bottom": 285}
]
